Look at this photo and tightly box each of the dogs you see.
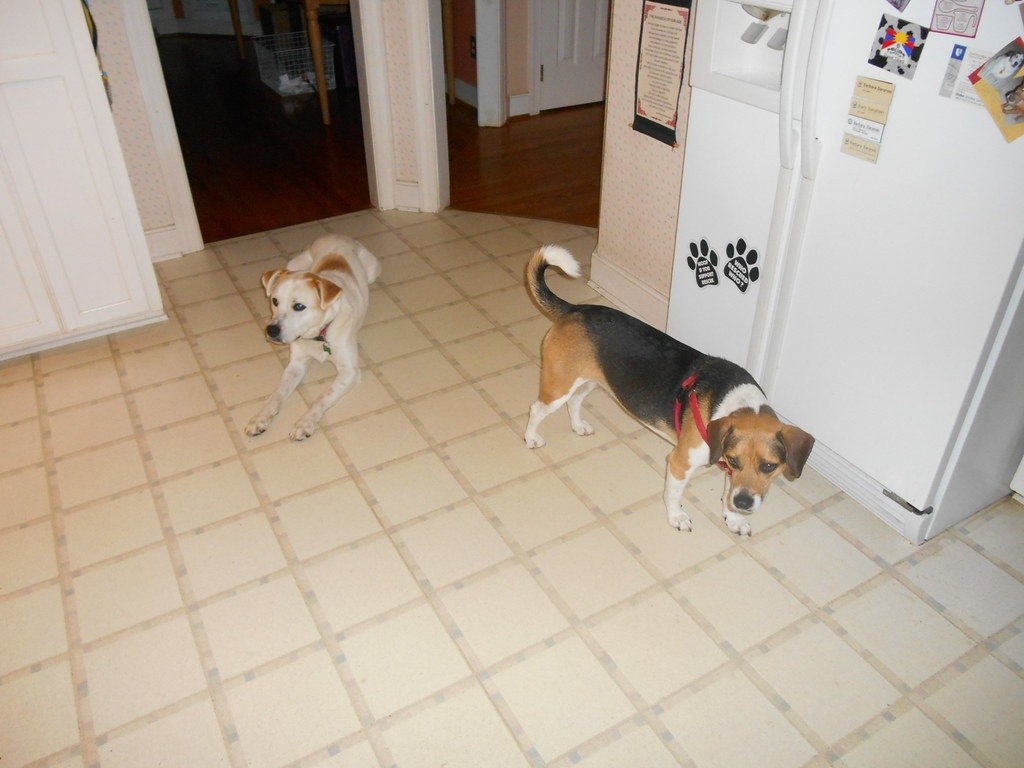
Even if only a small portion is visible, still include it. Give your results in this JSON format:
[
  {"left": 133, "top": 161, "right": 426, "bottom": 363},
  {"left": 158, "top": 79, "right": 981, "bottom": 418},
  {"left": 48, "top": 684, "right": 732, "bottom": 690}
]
[
  {"left": 523, "top": 244, "right": 815, "bottom": 538},
  {"left": 1001, "top": 84, "right": 1024, "bottom": 121},
  {"left": 244, "top": 232, "right": 382, "bottom": 442},
  {"left": 983, "top": 51, "right": 1024, "bottom": 86}
]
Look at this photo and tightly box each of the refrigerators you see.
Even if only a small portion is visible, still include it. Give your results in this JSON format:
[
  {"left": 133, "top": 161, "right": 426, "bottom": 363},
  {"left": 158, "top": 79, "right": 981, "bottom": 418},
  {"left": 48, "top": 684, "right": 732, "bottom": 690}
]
[{"left": 668, "top": 1, "right": 1023, "bottom": 544}]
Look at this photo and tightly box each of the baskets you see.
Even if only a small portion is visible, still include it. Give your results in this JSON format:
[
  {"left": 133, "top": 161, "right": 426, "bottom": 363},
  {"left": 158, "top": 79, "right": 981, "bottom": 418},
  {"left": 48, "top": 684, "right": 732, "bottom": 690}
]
[{"left": 253, "top": 31, "right": 337, "bottom": 97}]
